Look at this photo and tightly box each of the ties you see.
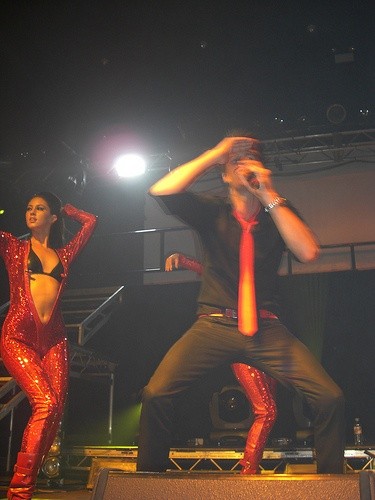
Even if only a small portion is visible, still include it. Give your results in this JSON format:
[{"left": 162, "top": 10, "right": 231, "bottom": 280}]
[{"left": 231, "top": 210, "right": 259, "bottom": 335}]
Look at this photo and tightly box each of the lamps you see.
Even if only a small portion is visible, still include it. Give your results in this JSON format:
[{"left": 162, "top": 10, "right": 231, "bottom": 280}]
[{"left": 269, "top": 103, "right": 350, "bottom": 136}]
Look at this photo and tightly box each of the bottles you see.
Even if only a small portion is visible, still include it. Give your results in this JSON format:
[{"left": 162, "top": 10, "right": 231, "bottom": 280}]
[{"left": 353, "top": 418, "right": 363, "bottom": 446}]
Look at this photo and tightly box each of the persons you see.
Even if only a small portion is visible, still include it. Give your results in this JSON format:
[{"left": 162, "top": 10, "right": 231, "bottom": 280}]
[
  {"left": 0, "top": 191, "right": 98, "bottom": 500},
  {"left": 165, "top": 252, "right": 277, "bottom": 474},
  {"left": 137, "top": 129, "right": 346, "bottom": 474}
]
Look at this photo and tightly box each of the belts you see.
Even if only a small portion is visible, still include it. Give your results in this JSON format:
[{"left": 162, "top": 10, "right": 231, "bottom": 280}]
[{"left": 199, "top": 308, "right": 279, "bottom": 321}]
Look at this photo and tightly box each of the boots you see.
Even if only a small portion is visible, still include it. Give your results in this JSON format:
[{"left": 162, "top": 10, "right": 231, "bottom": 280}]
[{"left": 8, "top": 452, "right": 40, "bottom": 499}]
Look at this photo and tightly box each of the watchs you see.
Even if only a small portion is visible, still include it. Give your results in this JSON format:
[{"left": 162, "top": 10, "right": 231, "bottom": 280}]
[{"left": 264, "top": 196, "right": 288, "bottom": 213}]
